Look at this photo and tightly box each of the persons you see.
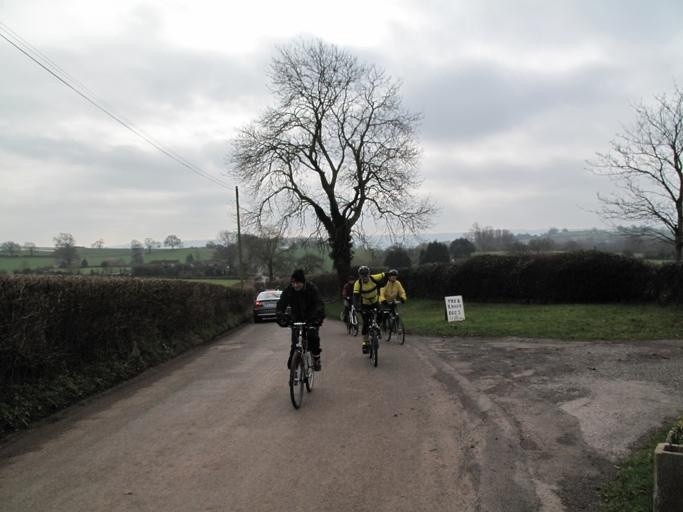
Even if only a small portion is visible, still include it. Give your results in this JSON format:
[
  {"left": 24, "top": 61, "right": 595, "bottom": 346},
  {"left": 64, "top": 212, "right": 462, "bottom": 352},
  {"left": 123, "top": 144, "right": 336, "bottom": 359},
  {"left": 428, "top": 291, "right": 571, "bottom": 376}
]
[
  {"left": 275, "top": 268, "right": 327, "bottom": 371},
  {"left": 342, "top": 275, "right": 357, "bottom": 324},
  {"left": 352, "top": 265, "right": 398, "bottom": 355},
  {"left": 379, "top": 268, "right": 407, "bottom": 332}
]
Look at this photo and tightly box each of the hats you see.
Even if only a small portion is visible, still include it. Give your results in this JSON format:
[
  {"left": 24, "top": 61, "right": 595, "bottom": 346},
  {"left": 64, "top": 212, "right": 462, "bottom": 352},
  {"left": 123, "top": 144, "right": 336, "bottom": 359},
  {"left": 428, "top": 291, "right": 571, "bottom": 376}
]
[
  {"left": 349, "top": 276, "right": 354, "bottom": 280},
  {"left": 291, "top": 269, "right": 305, "bottom": 282}
]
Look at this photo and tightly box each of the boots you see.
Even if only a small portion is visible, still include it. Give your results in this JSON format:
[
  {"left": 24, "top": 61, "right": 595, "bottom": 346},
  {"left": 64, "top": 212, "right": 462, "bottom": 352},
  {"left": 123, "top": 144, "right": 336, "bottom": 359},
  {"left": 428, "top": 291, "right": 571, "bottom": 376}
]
[
  {"left": 289, "top": 370, "right": 298, "bottom": 385},
  {"left": 312, "top": 353, "right": 320, "bottom": 371},
  {"left": 343, "top": 316, "right": 348, "bottom": 322}
]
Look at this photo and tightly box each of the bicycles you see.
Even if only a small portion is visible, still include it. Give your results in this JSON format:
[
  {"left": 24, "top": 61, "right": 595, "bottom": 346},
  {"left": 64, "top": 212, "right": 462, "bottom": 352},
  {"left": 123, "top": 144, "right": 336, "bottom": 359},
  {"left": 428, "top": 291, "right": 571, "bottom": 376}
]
[
  {"left": 381, "top": 301, "right": 404, "bottom": 346},
  {"left": 339, "top": 297, "right": 360, "bottom": 336},
  {"left": 283, "top": 320, "right": 319, "bottom": 409},
  {"left": 358, "top": 307, "right": 388, "bottom": 368}
]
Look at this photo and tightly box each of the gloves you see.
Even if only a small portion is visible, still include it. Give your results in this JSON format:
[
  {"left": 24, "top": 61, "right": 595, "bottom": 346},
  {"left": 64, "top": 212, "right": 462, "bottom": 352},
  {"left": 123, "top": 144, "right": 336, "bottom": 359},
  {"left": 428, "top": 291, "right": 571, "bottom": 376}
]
[
  {"left": 312, "top": 319, "right": 323, "bottom": 327},
  {"left": 276, "top": 312, "right": 288, "bottom": 327},
  {"left": 382, "top": 300, "right": 389, "bottom": 306},
  {"left": 354, "top": 304, "right": 360, "bottom": 310},
  {"left": 401, "top": 298, "right": 405, "bottom": 304},
  {"left": 378, "top": 276, "right": 388, "bottom": 286}
]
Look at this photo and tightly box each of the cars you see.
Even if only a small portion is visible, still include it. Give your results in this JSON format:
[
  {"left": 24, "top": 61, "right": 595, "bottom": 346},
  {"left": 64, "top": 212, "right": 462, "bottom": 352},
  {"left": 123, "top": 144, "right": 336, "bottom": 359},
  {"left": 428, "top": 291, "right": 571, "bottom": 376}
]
[{"left": 253, "top": 289, "right": 284, "bottom": 322}]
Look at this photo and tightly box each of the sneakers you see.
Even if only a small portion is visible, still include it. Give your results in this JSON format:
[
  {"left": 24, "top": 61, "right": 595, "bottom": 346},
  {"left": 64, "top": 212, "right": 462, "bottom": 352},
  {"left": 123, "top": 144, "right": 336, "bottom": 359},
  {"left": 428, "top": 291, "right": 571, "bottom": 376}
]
[{"left": 362, "top": 339, "right": 370, "bottom": 347}]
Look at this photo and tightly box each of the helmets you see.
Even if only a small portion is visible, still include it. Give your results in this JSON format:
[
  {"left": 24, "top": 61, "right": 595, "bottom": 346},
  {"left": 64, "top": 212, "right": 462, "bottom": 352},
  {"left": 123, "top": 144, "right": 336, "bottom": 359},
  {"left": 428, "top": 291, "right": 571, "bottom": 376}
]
[
  {"left": 357, "top": 266, "right": 370, "bottom": 275},
  {"left": 389, "top": 269, "right": 399, "bottom": 275}
]
